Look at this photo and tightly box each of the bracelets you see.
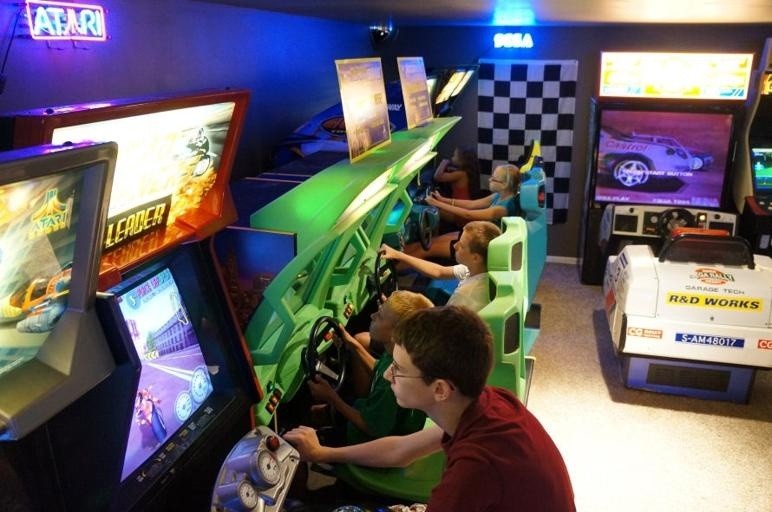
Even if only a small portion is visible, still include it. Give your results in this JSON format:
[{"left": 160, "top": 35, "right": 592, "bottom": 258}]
[{"left": 452, "top": 198, "right": 455, "bottom": 206}]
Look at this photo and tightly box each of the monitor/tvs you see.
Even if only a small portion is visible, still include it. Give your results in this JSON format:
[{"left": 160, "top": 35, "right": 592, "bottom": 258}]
[
  {"left": 749, "top": 142, "right": 772, "bottom": 197},
  {"left": 592, "top": 103, "right": 738, "bottom": 211},
  {"left": 104, "top": 260, "right": 212, "bottom": 491}
]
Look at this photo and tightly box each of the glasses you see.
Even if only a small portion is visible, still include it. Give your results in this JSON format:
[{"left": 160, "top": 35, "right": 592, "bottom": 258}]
[
  {"left": 391, "top": 360, "right": 454, "bottom": 390},
  {"left": 489, "top": 177, "right": 504, "bottom": 184}
]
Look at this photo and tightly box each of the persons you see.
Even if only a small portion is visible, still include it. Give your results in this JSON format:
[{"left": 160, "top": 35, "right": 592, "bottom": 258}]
[
  {"left": 283, "top": 303, "right": 576, "bottom": 511},
  {"left": 310, "top": 290, "right": 435, "bottom": 446},
  {"left": 395, "top": 164, "right": 522, "bottom": 271},
  {"left": 348, "top": 220, "right": 501, "bottom": 398},
  {"left": 434, "top": 143, "right": 474, "bottom": 200}
]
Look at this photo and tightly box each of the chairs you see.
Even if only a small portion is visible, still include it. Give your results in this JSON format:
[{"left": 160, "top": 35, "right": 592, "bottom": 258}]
[
  {"left": 343, "top": 270, "right": 529, "bottom": 498},
  {"left": 658, "top": 227, "right": 754, "bottom": 266},
  {"left": 414, "top": 213, "right": 533, "bottom": 325},
  {"left": 425, "top": 166, "right": 551, "bottom": 353},
  {"left": 516, "top": 137, "right": 542, "bottom": 173}
]
